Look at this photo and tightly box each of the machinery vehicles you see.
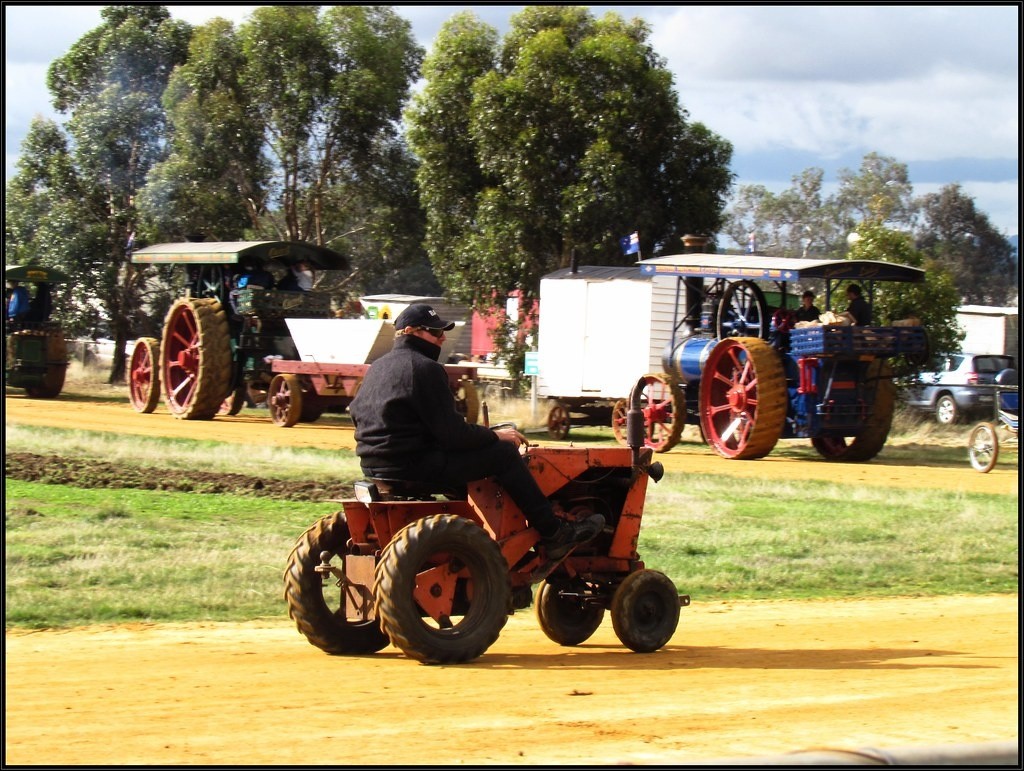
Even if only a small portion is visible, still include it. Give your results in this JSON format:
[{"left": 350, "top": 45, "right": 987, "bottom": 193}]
[
  {"left": 122, "top": 238, "right": 361, "bottom": 421},
  {"left": 3, "top": 263, "right": 75, "bottom": 398},
  {"left": 281, "top": 376, "right": 692, "bottom": 666},
  {"left": 624, "top": 251, "right": 933, "bottom": 465}
]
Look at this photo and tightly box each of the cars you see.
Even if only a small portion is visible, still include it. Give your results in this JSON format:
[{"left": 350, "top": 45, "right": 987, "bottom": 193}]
[{"left": 895, "top": 349, "right": 1018, "bottom": 428}]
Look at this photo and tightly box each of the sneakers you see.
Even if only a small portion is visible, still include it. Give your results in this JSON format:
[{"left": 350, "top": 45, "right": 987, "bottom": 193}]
[{"left": 541, "top": 513, "right": 605, "bottom": 560}]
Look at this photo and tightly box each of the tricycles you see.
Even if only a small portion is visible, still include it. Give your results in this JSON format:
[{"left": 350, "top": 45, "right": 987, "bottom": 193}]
[{"left": 965, "top": 369, "right": 1019, "bottom": 473}]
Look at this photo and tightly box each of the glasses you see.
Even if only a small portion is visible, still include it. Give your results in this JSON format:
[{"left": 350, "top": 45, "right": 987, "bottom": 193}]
[{"left": 410, "top": 325, "right": 443, "bottom": 337}]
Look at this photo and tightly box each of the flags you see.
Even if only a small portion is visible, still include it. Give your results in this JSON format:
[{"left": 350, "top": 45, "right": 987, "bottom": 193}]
[
  {"left": 127, "top": 232, "right": 135, "bottom": 248},
  {"left": 621, "top": 233, "right": 640, "bottom": 256}
]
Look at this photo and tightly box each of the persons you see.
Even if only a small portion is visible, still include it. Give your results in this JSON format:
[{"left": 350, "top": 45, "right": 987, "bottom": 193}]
[
  {"left": 845, "top": 282, "right": 871, "bottom": 326},
  {"left": 226, "top": 259, "right": 297, "bottom": 291},
  {"left": 293, "top": 263, "right": 314, "bottom": 291},
  {"left": 794, "top": 292, "right": 820, "bottom": 325},
  {"left": 350, "top": 304, "right": 605, "bottom": 559},
  {"left": 6, "top": 281, "right": 52, "bottom": 332}
]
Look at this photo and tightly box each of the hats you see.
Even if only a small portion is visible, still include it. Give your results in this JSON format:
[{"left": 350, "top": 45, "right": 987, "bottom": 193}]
[
  {"left": 394, "top": 303, "right": 455, "bottom": 333},
  {"left": 802, "top": 292, "right": 815, "bottom": 298}
]
